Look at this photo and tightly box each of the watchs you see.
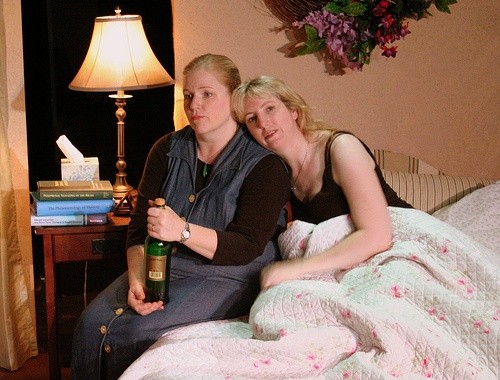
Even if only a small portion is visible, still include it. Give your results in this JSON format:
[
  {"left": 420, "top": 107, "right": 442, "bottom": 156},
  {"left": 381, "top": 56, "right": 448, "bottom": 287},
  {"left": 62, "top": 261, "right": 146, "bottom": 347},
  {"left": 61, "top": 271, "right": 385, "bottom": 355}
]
[{"left": 179, "top": 222, "right": 191, "bottom": 244}]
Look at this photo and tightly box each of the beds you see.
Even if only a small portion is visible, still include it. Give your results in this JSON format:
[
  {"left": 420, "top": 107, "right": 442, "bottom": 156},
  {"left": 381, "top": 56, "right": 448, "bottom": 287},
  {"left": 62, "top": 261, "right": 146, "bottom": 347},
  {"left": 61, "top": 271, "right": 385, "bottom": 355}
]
[{"left": 119, "top": 170, "right": 500, "bottom": 380}]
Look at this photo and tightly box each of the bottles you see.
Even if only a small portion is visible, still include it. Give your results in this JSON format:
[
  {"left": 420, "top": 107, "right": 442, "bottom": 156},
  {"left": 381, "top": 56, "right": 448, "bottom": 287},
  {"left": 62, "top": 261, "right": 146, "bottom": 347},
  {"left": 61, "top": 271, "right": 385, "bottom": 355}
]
[{"left": 143, "top": 198, "right": 170, "bottom": 306}]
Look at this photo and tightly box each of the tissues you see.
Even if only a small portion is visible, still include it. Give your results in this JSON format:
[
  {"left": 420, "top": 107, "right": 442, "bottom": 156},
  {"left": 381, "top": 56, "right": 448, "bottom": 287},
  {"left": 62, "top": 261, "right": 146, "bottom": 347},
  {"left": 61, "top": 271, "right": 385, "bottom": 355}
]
[{"left": 55, "top": 134, "right": 100, "bottom": 181}]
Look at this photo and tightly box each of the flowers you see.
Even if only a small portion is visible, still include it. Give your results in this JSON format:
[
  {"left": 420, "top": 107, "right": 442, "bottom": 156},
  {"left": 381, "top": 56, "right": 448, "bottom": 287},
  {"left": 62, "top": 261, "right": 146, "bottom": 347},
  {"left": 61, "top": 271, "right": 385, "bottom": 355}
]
[{"left": 291, "top": 0, "right": 457, "bottom": 71}]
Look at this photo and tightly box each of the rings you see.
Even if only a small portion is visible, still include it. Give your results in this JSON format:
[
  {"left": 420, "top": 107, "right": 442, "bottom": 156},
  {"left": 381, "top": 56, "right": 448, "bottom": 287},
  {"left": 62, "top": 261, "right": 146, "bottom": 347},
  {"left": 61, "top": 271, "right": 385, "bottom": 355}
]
[{"left": 152, "top": 225, "right": 154, "bottom": 232}]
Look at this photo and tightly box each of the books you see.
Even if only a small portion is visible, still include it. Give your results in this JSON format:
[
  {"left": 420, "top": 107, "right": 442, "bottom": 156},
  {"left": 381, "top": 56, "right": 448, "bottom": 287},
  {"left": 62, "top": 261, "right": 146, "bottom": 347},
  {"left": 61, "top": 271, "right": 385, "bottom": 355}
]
[{"left": 29, "top": 181, "right": 114, "bottom": 226}]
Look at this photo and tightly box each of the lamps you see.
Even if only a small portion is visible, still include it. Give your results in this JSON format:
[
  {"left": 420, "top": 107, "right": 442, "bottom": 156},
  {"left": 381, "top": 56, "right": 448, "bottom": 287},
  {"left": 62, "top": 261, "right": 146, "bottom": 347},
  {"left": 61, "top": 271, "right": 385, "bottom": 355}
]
[{"left": 69, "top": 7, "right": 177, "bottom": 198}]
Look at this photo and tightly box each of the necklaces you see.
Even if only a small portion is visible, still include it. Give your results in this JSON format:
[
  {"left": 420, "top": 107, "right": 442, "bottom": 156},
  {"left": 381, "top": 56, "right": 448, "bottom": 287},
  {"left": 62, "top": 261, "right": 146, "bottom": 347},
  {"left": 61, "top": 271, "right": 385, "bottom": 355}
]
[
  {"left": 200, "top": 150, "right": 219, "bottom": 177},
  {"left": 290, "top": 151, "right": 308, "bottom": 191}
]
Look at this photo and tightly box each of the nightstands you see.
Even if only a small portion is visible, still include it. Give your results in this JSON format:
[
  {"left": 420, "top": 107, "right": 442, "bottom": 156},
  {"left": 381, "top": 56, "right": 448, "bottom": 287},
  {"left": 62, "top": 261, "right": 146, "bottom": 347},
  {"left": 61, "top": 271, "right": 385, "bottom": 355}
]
[{"left": 34, "top": 212, "right": 131, "bottom": 380}]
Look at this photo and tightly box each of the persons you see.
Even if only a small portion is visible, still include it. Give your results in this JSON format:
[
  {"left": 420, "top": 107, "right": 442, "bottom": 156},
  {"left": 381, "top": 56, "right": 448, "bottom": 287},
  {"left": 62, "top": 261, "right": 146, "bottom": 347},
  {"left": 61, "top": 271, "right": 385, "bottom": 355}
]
[
  {"left": 73, "top": 53, "right": 294, "bottom": 380},
  {"left": 230, "top": 75, "right": 415, "bottom": 292}
]
